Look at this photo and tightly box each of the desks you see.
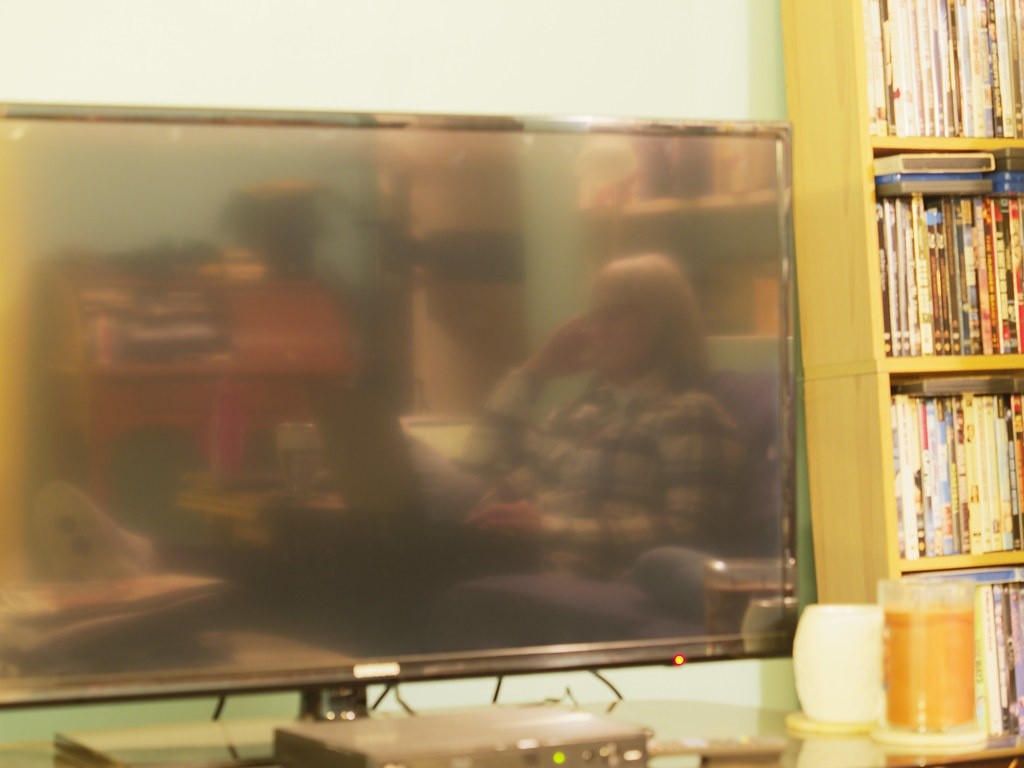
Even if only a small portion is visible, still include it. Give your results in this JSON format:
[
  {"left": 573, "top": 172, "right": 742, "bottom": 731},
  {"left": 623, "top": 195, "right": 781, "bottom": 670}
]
[{"left": 0, "top": 699, "right": 1024, "bottom": 768}]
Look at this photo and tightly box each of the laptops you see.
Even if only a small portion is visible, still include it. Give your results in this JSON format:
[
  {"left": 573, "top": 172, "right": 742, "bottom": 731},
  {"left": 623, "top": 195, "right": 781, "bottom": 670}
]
[{"left": 305, "top": 376, "right": 538, "bottom": 554}]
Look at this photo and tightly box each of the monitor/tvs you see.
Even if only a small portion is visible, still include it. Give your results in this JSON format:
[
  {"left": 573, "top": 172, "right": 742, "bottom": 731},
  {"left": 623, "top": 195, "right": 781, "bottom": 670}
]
[{"left": 0, "top": 103, "right": 818, "bottom": 722}]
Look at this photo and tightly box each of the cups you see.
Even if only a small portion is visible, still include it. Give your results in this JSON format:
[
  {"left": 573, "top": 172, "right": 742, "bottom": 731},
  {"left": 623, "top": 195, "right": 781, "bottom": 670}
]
[
  {"left": 878, "top": 578, "right": 991, "bottom": 753},
  {"left": 792, "top": 604, "right": 883, "bottom": 724}
]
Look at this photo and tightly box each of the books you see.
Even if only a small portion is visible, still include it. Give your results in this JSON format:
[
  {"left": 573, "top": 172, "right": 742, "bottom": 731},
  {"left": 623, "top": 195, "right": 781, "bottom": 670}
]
[
  {"left": 890, "top": 373, "right": 1024, "bottom": 559},
  {"left": 875, "top": 153, "right": 1024, "bottom": 357},
  {"left": 903, "top": 565, "right": 1024, "bottom": 747},
  {"left": 867, "top": 0, "right": 1024, "bottom": 141}
]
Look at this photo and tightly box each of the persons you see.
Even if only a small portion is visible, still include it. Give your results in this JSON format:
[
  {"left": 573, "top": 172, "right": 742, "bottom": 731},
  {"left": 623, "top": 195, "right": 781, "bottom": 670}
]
[{"left": 255, "top": 255, "right": 758, "bottom": 641}]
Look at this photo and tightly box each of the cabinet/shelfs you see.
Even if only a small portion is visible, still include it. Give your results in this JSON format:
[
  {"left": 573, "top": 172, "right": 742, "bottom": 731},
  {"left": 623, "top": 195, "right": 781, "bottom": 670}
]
[{"left": 782, "top": 0, "right": 1024, "bottom": 743}]
